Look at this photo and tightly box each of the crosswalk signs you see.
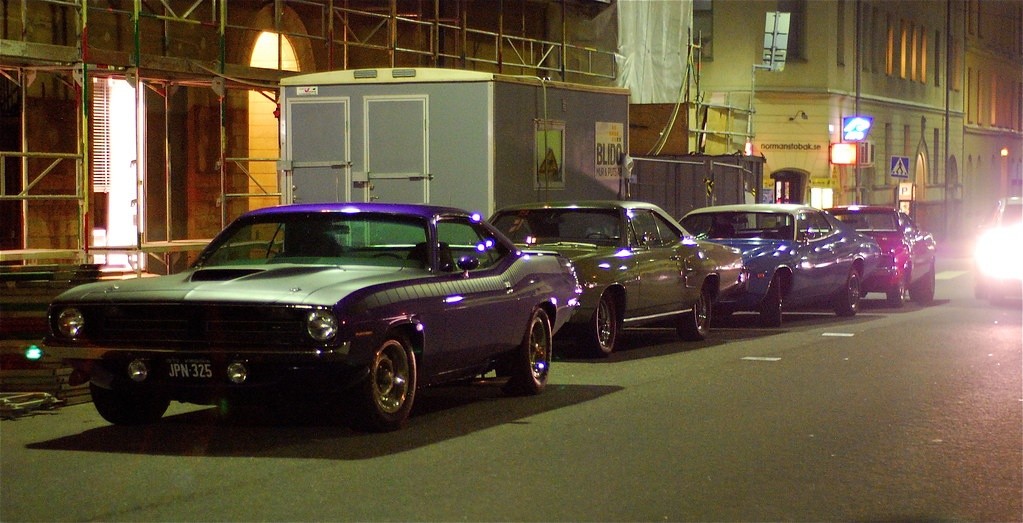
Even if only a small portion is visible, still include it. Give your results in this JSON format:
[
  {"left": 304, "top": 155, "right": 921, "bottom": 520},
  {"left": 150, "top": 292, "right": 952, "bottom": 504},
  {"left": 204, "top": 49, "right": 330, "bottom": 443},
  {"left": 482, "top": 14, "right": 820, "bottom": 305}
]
[{"left": 890, "top": 155, "right": 908, "bottom": 179}]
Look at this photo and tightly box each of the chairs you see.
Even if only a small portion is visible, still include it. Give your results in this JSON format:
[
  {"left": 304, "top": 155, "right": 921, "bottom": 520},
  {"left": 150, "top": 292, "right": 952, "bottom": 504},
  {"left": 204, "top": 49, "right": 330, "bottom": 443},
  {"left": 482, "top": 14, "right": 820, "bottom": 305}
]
[
  {"left": 404, "top": 241, "right": 459, "bottom": 273},
  {"left": 275, "top": 232, "right": 341, "bottom": 258}
]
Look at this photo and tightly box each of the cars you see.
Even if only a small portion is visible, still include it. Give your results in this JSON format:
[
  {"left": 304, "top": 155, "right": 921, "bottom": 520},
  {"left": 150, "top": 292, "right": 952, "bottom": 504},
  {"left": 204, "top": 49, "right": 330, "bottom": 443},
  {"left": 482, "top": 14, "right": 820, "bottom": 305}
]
[
  {"left": 673, "top": 204, "right": 880, "bottom": 329},
  {"left": 975, "top": 197, "right": 1023, "bottom": 304},
  {"left": 42, "top": 203, "right": 582, "bottom": 432},
  {"left": 476, "top": 201, "right": 746, "bottom": 358},
  {"left": 818, "top": 207, "right": 937, "bottom": 310}
]
